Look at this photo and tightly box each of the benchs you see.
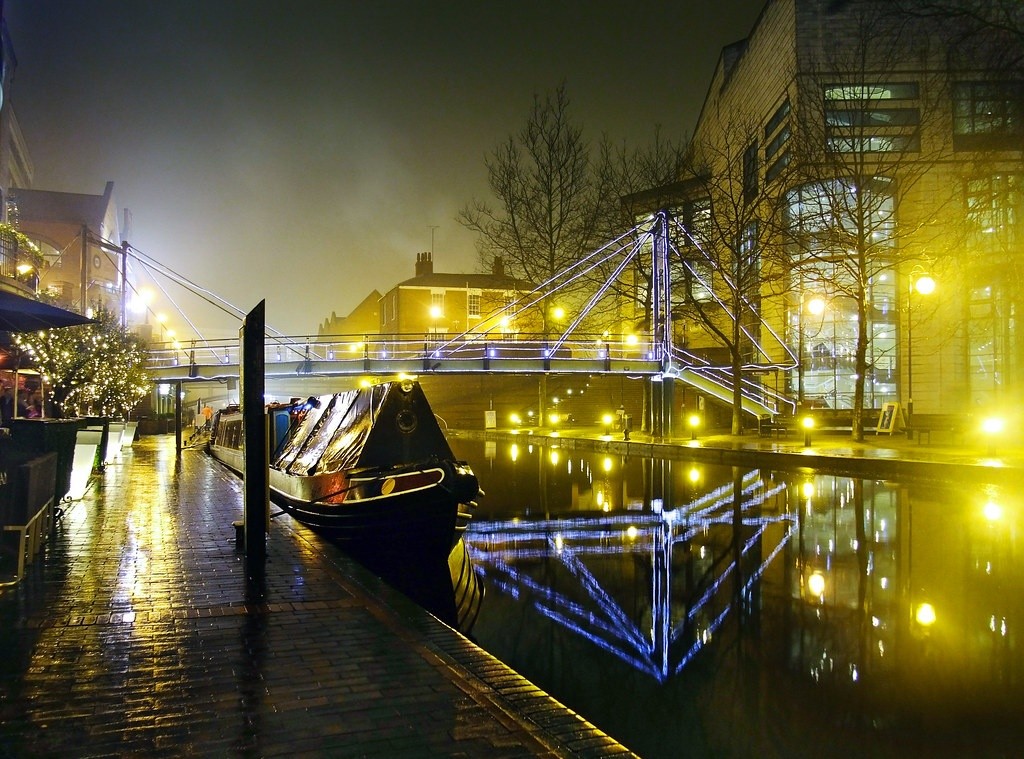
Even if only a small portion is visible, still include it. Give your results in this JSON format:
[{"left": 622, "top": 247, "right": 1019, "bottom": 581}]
[
  {"left": 900, "top": 414, "right": 978, "bottom": 446},
  {"left": 762, "top": 414, "right": 802, "bottom": 439}
]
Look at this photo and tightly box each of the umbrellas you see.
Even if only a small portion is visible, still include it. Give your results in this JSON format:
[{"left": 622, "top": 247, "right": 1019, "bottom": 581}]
[{"left": 0, "top": 289, "right": 101, "bottom": 331}]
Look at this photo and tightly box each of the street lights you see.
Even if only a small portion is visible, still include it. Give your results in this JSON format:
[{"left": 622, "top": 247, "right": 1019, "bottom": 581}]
[
  {"left": 794, "top": 268, "right": 824, "bottom": 431},
  {"left": 903, "top": 266, "right": 937, "bottom": 441}
]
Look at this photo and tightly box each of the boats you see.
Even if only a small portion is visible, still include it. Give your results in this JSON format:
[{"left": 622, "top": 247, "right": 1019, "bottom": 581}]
[{"left": 207, "top": 378, "right": 479, "bottom": 540}]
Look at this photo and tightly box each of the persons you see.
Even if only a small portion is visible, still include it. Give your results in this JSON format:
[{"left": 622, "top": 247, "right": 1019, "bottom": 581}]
[
  {"left": 0, "top": 374, "right": 59, "bottom": 427},
  {"left": 201, "top": 405, "right": 214, "bottom": 421}
]
